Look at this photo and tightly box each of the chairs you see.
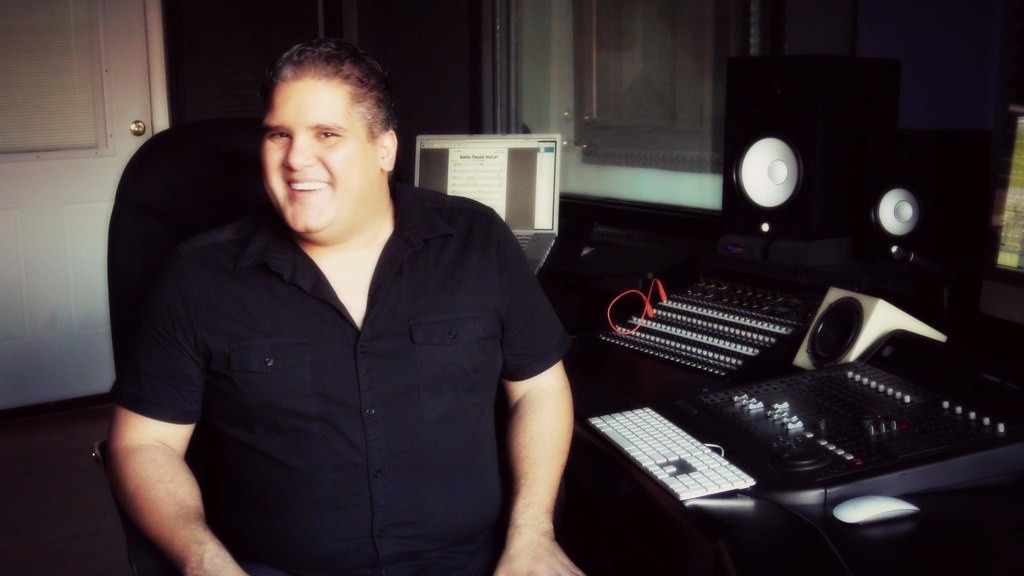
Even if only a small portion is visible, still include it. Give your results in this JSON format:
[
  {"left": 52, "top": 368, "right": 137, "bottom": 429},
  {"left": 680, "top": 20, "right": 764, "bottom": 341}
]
[{"left": 91, "top": 118, "right": 401, "bottom": 575}]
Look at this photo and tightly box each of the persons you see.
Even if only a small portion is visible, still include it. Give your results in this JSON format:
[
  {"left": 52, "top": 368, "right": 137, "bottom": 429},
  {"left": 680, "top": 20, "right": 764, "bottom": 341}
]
[{"left": 110, "top": 43, "right": 586, "bottom": 576}]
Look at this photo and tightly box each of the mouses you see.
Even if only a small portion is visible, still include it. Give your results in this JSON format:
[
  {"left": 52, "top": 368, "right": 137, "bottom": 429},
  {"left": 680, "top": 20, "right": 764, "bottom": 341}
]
[{"left": 833, "top": 495, "right": 919, "bottom": 525}]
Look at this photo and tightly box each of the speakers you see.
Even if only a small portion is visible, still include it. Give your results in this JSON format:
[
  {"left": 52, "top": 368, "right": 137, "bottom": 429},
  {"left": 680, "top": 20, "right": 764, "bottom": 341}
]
[
  {"left": 725, "top": 53, "right": 901, "bottom": 241},
  {"left": 875, "top": 130, "right": 1000, "bottom": 332}
]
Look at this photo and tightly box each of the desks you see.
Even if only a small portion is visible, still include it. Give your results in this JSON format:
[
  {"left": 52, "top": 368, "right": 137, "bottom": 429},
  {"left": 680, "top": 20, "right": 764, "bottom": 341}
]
[{"left": 489, "top": 236, "right": 1024, "bottom": 576}]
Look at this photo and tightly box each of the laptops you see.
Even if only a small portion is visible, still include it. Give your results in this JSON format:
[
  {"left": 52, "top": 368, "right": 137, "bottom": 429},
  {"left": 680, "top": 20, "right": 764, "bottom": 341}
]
[{"left": 414, "top": 133, "right": 561, "bottom": 275}]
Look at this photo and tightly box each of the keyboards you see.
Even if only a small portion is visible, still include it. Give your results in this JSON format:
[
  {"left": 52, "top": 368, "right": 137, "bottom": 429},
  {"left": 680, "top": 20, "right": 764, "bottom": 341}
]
[{"left": 587, "top": 406, "right": 756, "bottom": 501}]
